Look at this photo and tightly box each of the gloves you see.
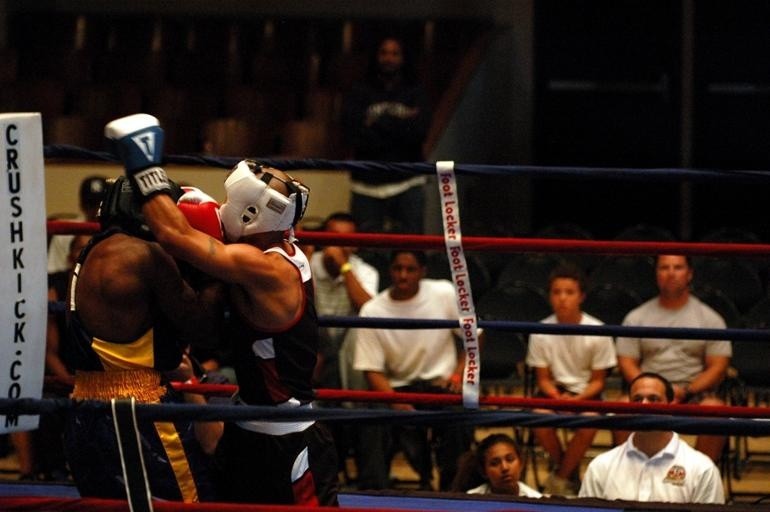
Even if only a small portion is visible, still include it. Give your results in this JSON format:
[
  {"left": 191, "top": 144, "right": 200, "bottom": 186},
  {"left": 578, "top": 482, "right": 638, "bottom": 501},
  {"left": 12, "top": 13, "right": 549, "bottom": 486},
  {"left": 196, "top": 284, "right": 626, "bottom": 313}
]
[{"left": 103, "top": 112, "right": 173, "bottom": 209}]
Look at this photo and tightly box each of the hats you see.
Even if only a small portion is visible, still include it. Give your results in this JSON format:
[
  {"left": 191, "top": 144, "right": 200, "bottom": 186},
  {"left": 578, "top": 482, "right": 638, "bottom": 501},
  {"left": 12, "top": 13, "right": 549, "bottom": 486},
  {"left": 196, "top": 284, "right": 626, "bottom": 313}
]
[{"left": 77, "top": 176, "right": 106, "bottom": 217}]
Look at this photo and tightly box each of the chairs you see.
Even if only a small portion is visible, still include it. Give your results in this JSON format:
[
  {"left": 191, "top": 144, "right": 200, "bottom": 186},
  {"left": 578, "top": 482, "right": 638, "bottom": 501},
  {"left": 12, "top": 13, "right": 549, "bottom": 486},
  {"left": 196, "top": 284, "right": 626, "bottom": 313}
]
[{"left": 522, "top": 364, "right": 752, "bottom": 497}]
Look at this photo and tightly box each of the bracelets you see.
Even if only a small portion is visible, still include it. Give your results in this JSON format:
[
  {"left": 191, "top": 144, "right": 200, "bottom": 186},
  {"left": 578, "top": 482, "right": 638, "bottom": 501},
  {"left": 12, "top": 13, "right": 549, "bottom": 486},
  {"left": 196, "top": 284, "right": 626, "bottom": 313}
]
[{"left": 679, "top": 382, "right": 695, "bottom": 403}]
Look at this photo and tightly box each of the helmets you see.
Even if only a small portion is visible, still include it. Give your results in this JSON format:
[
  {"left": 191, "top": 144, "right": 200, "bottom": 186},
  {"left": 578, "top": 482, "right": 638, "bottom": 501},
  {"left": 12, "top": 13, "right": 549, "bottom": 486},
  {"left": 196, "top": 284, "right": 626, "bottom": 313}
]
[
  {"left": 221, "top": 158, "right": 311, "bottom": 237},
  {"left": 97, "top": 173, "right": 184, "bottom": 241}
]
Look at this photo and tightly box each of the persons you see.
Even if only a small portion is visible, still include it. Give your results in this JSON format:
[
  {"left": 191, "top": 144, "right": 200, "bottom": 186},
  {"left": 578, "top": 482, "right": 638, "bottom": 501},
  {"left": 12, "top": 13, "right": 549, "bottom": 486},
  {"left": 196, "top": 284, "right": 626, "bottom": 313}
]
[
  {"left": 106, "top": 114, "right": 342, "bottom": 507},
  {"left": 172, "top": 291, "right": 241, "bottom": 499},
  {"left": 610, "top": 252, "right": 732, "bottom": 465},
  {"left": 465, "top": 432, "right": 543, "bottom": 499},
  {"left": 297, "top": 217, "right": 480, "bottom": 490},
  {"left": 344, "top": 36, "right": 427, "bottom": 238},
  {"left": 522, "top": 267, "right": 619, "bottom": 497},
  {"left": 67, "top": 174, "right": 222, "bottom": 505},
  {"left": 13, "top": 176, "right": 112, "bottom": 483},
  {"left": 577, "top": 371, "right": 726, "bottom": 505}
]
